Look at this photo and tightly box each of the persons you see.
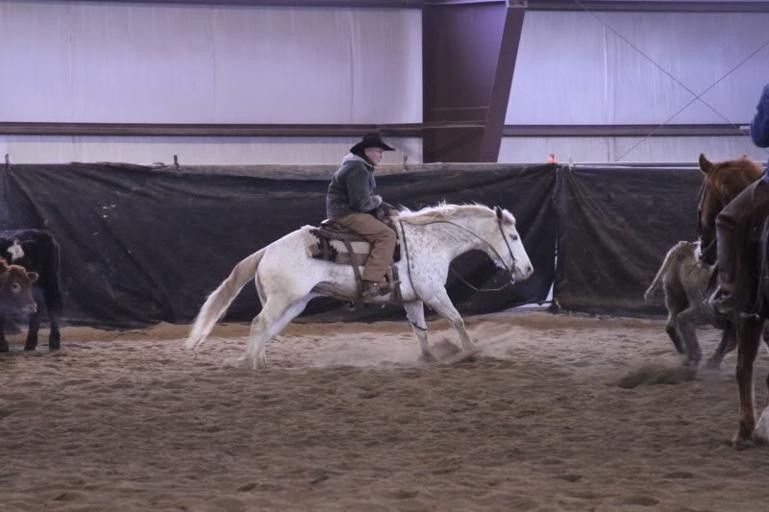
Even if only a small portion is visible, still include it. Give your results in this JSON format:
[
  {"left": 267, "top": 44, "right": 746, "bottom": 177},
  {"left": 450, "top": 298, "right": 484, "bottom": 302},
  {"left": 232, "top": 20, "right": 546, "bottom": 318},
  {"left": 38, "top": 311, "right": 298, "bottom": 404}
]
[
  {"left": 699, "top": 83, "right": 768, "bottom": 317},
  {"left": 324, "top": 129, "right": 400, "bottom": 303},
  {"left": 0, "top": 224, "right": 63, "bottom": 354}
]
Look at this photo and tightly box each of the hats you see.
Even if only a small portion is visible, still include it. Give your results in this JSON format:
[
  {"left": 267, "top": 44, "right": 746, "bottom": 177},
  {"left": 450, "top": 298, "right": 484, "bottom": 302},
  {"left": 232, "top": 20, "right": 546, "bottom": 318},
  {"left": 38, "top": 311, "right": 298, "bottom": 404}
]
[{"left": 350, "top": 133, "right": 395, "bottom": 155}]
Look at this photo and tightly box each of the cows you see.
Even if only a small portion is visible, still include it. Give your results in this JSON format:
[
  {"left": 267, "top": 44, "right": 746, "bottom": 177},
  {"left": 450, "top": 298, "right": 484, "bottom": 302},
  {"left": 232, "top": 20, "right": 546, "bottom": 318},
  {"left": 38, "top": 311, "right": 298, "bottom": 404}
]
[
  {"left": 643, "top": 237, "right": 739, "bottom": 369},
  {"left": 0, "top": 226, "right": 62, "bottom": 353}
]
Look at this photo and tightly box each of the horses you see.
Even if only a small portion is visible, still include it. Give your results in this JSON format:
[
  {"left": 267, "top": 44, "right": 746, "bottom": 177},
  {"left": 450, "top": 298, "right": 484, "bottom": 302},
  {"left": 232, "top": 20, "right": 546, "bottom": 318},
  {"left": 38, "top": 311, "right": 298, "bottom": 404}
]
[
  {"left": 691, "top": 151, "right": 769, "bottom": 451},
  {"left": 184, "top": 198, "right": 536, "bottom": 371}
]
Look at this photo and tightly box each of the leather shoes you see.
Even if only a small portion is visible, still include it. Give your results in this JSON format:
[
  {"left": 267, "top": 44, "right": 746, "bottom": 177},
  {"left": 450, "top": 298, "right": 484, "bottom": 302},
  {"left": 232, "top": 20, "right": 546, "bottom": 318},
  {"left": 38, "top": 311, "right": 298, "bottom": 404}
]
[
  {"left": 702, "top": 293, "right": 735, "bottom": 314},
  {"left": 359, "top": 280, "right": 394, "bottom": 299}
]
